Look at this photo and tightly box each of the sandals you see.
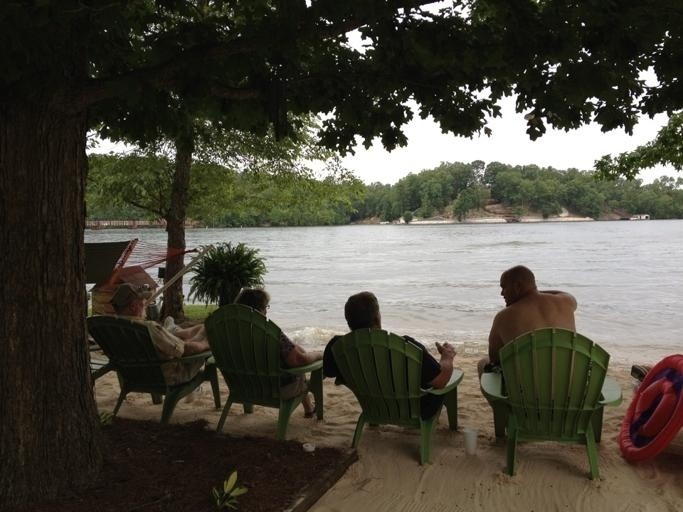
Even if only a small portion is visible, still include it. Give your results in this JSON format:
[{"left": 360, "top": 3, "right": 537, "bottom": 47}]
[{"left": 304, "top": 400, "right": 317, "bottom": 419}]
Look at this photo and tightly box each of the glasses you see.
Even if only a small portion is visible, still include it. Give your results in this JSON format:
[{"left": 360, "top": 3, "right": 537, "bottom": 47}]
[{"left": 261, "top": 304, "right": 271, "bottom": 309}]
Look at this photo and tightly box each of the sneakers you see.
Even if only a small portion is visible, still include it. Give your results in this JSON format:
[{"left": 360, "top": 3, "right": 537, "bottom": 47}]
[
  {"left": 163, "top": 314, "right": 179, "bottom": 336},
  {"left": 185, "top": 384, "right": 207, "bottom": 404}
]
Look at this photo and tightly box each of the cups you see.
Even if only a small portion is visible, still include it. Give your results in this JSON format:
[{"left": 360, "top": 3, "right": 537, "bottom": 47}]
[{"left": 462, "top": 428, "right": 478, "bottom": 455}]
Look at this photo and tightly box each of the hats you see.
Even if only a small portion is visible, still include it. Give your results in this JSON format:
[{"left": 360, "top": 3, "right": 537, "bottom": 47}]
[{"left": 108, "top": 282, "right": 153, "bottom": 312}]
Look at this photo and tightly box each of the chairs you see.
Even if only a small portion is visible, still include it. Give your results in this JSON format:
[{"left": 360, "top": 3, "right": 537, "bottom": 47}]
[
  {"left": 319, "top": 325, "right": 464, "bottom": 465},
  {"left": 79, "top": 308, "right": 225, "bottom": 422},
  {"left": 477, "top": 324, "right": 624, "bottom": 486},
  {"left": 202, "top": 294, "right": 328, "bottom": 436}
]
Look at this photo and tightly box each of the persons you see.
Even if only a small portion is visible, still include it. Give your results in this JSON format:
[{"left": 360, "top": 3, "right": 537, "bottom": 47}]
[
  {"left": 321, "top": 290, "right": 458, "bottom": 420},
  {"left": 232, "top": 286, "right": 325, "bottom": 418},
  {"left": 109, "top": 281, "right": 212, "bottom": 386},
  {"left": 476, "top": 265, "right": 578, "bottom": 383}
]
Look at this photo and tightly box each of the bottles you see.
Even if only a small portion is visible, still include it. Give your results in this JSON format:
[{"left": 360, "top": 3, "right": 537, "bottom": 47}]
[{"left": 302, "top": 443, "right": 315, "bottom": 480}]
